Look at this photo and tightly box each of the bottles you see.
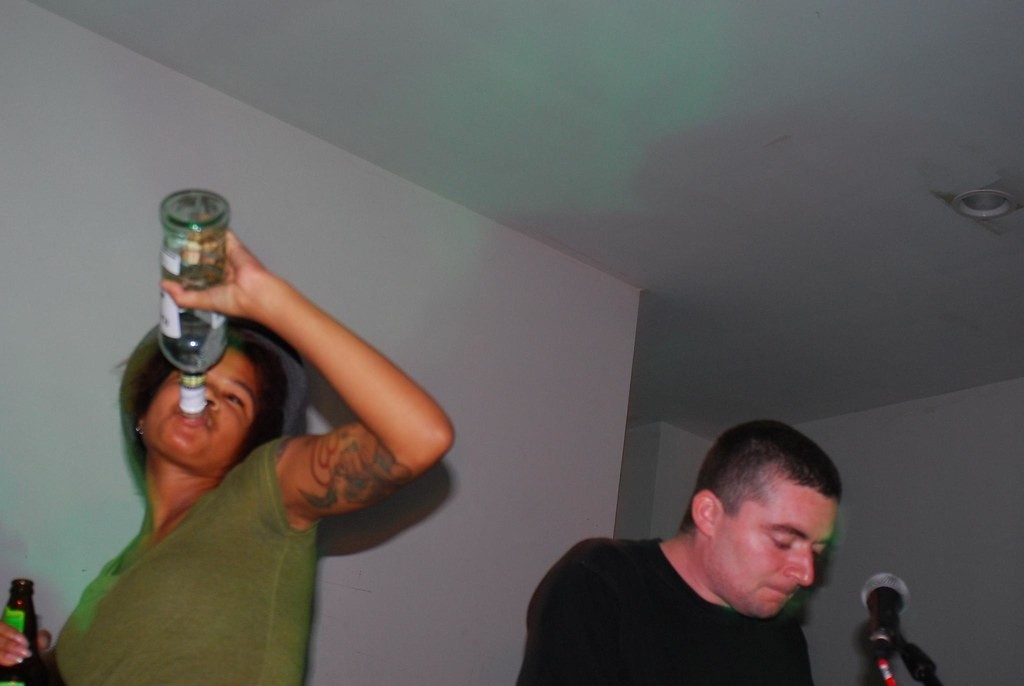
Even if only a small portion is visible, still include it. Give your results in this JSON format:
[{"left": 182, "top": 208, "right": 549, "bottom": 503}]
[
  {"left": 158, "top": 188, "right": 230, "bottom": 418},
  {"left": 0, "top": 579, "right": 47, "bottom": 686}
]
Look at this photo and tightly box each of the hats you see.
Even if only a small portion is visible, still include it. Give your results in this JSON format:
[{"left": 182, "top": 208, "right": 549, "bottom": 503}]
[{"left": 122, "top": 320, "right": 307, "bottom": 462}]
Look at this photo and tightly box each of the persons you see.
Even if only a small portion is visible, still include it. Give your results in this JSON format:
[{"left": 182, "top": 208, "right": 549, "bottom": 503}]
[
  {"left": 0, "top": 214, "right": 457, "bottom": 686},
  {"left": 514, "top": 418, "right": 843, "bottom": 686}
]
